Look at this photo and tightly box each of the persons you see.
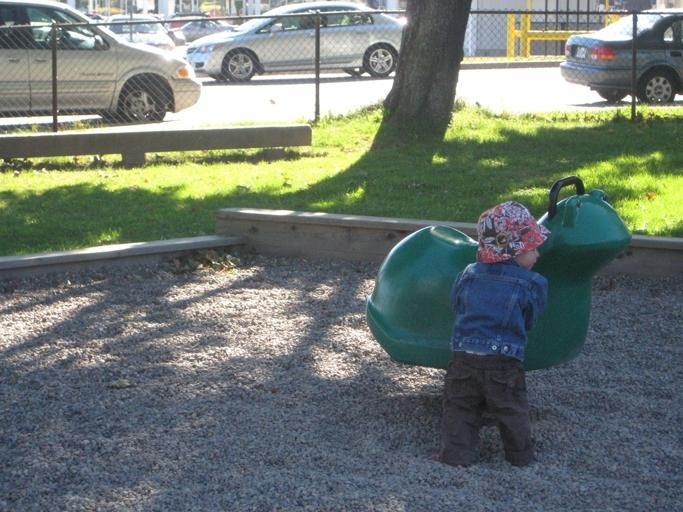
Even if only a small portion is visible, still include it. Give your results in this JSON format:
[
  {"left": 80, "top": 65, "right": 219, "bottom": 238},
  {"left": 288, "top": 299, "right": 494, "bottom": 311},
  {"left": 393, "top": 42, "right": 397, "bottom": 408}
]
[{"left": 431, "top": 198, "right": 550, "bottom": 466}]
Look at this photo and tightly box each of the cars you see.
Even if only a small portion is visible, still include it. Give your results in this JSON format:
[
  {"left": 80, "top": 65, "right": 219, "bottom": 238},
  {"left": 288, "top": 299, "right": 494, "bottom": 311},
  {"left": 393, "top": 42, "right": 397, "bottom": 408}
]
[
  {"left": 105, "top": 13, "right": 174, "bottom": 50},
  {"left": 559, "top": 7, "right": 682, "bottom": 106},
  {"left": 187, "top": 3, "right": 405, "bottom": 83},
  {"left": 152, "top": 11, "right": 240, "bottom": 46},
  {"left": 1, "top": 1, "right": 203, "bottom": 127}
]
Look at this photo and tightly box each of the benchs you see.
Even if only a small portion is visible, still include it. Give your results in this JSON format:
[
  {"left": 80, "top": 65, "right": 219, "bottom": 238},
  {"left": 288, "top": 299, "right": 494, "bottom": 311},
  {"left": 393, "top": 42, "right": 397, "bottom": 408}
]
[{"left": 0, "top": 122, "right": 312, "bottom": 170}]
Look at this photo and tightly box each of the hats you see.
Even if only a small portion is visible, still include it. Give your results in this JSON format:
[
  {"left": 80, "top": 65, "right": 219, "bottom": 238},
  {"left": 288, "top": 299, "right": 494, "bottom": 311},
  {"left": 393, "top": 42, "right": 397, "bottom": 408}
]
[{"left": 474, "top": 200, "right": 551, "bottom": 265}]
